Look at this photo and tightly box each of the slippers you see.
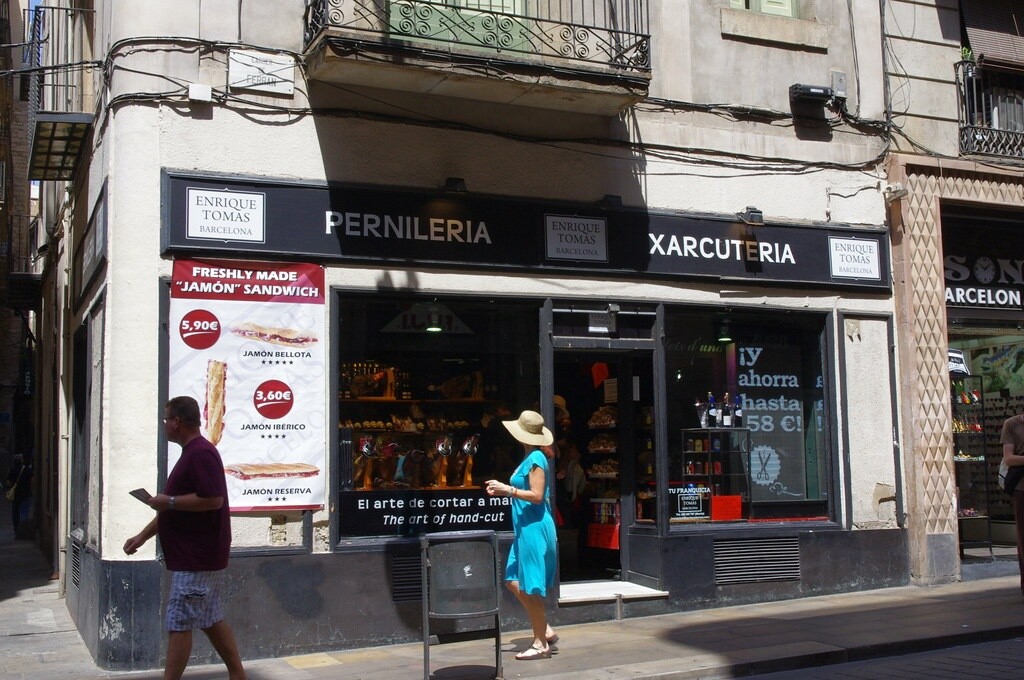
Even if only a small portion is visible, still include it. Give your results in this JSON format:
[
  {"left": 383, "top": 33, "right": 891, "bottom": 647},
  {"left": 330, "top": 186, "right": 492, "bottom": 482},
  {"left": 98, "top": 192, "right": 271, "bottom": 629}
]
[
  {"left": 547, "top": 635, "right": 559, "bottom": 645},
  {"left": 516, "top": 645, "right": 551, "bottom": 659}
]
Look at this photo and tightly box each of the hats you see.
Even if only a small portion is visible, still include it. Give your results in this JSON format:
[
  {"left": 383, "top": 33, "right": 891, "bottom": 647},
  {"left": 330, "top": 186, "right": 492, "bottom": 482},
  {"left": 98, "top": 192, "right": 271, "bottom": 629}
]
[
  {"left": 554, "top": 395, "right": 569, "bottom": 418},
  {"left": 502, "top": 410, "right": 554, "bottom": 446}
]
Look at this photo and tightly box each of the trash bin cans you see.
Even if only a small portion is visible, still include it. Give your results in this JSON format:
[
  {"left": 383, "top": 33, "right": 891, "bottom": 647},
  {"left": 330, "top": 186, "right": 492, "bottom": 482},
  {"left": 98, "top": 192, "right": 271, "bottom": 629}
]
[{"left": 420, "top": 529, "right": 503, "bottom": 680}]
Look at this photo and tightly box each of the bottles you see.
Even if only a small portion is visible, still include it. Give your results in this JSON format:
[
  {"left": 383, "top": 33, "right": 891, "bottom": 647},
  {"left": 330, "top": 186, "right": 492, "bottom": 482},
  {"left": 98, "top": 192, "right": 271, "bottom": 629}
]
[
  {"left": 695, "top": 392, "right": 733, "bottom": 427},
  {"left": 589, "top": 478, "right": 621, "bottom": 524},
  {"left": 686, "top": 436, "right": 722, "bottom": 498},
  {"left": 395, "top": 369, "right": 412, "bottom": 399},
  {"left": 636, "top": 500, "right": 658, "bottom": 519}
]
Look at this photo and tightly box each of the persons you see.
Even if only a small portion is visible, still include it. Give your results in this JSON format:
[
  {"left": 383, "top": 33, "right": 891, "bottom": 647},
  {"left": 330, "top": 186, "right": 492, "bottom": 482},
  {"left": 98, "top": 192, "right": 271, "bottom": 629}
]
[
  {"left": 6, "top": 454, "right": 34, "bottom": 540},
  {"left": 554, "top": 394, "right": 581, "bottom": 579},
  {"left": 168, "top": 496, "right": 175, "bottom": 510},
  {"left": 123, "top": 396, "right": 248, "bottom": 680},
  {"left": 1000, "top": 412, "right": 1024, "bottom": 597},
  {"left": 483, "top": 409, "right": 560, "bottom": 661}
]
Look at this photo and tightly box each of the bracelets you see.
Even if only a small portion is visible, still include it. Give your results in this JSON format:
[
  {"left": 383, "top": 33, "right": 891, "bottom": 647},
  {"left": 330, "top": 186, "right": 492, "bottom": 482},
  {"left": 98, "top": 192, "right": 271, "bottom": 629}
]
[
  {"left": 506, "top": 486, "right": 514, "bottom": 497},
  {"left": 515, "top": 487, "right": 517, "bottom": 498}
]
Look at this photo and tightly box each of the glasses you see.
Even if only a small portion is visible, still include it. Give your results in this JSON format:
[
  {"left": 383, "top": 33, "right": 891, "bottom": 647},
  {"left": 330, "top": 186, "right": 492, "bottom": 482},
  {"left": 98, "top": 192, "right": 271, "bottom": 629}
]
[{"left": 162, "top": 417, "right": 174, "bottom": 424}]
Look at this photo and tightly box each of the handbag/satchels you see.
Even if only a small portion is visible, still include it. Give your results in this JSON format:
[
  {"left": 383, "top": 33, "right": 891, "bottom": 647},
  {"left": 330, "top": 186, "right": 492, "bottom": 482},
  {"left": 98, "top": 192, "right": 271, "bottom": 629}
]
[
  {"left": 998, "top": 460, "right": 1024, "bottom": 494},
  {"left": 6, "top": 487, "right": 15, "bottom": 501}
]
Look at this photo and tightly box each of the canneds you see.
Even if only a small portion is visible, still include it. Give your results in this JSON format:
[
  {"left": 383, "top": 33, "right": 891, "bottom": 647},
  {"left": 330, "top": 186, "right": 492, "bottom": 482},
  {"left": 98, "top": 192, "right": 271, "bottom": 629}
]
[{"left": 685, "top": 439, "right": 722, "bottom": 498}]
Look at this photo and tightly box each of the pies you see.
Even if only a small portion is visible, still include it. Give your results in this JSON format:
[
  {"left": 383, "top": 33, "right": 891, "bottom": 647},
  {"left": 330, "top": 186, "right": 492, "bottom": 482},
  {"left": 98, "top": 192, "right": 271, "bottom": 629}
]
[
  {"left": 228, "top": 324, "right": 318, "bottom": 348},
  {"left": 204, "top": 360, "right": 226, "bottom": 445},
  {"left": 224, "top": 464, "right": 320, "bottom": 479}
]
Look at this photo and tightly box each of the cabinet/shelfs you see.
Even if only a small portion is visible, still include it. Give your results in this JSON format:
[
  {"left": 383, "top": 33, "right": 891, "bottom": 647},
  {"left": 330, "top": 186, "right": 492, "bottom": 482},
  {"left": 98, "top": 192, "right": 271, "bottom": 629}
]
[
  {"left": 582, "top": 421, "right": 624, "bottom": 550},
  {"left": 354, "top": 395, "right": 497, "bottom": 435},
  {"left": 678, "top": 425, "right": 753, "bottom": 519},
  {"left": 632, "top": 400, "right": 657, "bottom": 524},
  {"left": 950, "top": 372, "right": 998, "bottom": 562}
]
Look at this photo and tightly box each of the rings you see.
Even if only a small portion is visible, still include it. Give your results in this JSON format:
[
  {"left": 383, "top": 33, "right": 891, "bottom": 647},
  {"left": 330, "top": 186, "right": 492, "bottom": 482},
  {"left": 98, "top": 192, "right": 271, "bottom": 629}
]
[{"left": 492, "top": 484, "right": 493, "bottom": 490}]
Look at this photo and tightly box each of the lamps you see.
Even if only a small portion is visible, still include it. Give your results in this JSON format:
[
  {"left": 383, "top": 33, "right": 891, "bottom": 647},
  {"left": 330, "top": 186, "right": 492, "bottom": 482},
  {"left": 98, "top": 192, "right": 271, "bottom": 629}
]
[
  {"left": 789, "top": 83, "right": 835, "bottom": 105},
  {"left": 425, "top": 310, "right": 443, "bottom": 333},
  {"left": 597, "top": 193, "right": 625, "bottom": 213},
  {"left": 734, "top": 206, "right": 766, "bottom": 227},
  {"left": 606, "top": 302, "right": 622, "bottom": 314},
  {"left": 716, "top": 325, "right": 734, "bottom": 343},
  {"left": 440, "top": 176, "right": 468, "bottom": 198}
]
